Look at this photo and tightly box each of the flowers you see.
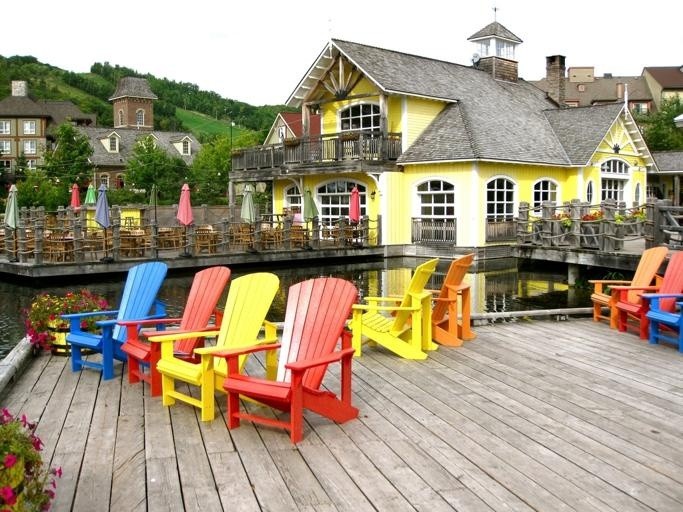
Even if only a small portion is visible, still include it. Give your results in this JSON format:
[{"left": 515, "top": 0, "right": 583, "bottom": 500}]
[
  {"left": 20, "top": 288, "right": 113, "bottom": 356},
  {"left": 0, "top": 408, "right": 62, "bottom": 512}
]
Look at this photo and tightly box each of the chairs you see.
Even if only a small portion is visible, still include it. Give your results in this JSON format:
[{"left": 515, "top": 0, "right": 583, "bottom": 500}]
[
  {"left": 588, "top": 246, "right": 683, "bottom": 354},
  {"left": 148, "top": 272, "right": 280, "bottom": 422},
  {"left": 116, "top": 266, "right": 231, "bottom": 397},
  {"left": 59, "top": 263, "right": 168, "bottom": 380},
  {"left": 211, "top": 277, "right": 359, "bottom": 445},
  {"left": 348, "top": 257, "right": 440, "bottom": 362},
  {"left": 390, "top": 254, "right": 477, "bottom": 347},
  {"left": 0, "top": 221, "right": 363, "bottom": 263}
]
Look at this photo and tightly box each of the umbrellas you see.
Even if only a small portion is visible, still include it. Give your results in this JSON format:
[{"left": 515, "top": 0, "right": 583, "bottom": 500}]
[
  {"left": 303, "top": 184, "right": 320, "bottom": 248},
  {"left": 69, "top": 183, "right": 80, "bottom": 217},
  {"left": 349, "top": 187, "right": 361, "bottom": 245},
  {"left": 92, "top": 182, "right": 110, "bottom": 259},
  {"left": 141, "top": 183, "right": 163, "bottom": 258},
  {"left": 240, "top": 184, "right": 255, "bottom": 250},
  {"left": 176, "top": 184, "right": 195, "bottom": 254},
  {"left": 3, "top": 184, "right": 20, "bottom": 261},
  {"left": 82, "top": 184, "right": 100, "bottom": 236}
]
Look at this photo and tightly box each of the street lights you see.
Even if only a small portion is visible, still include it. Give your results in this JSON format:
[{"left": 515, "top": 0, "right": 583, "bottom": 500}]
[{"left": 230, "top": 121, "right": 235, "bottom": 171}]
